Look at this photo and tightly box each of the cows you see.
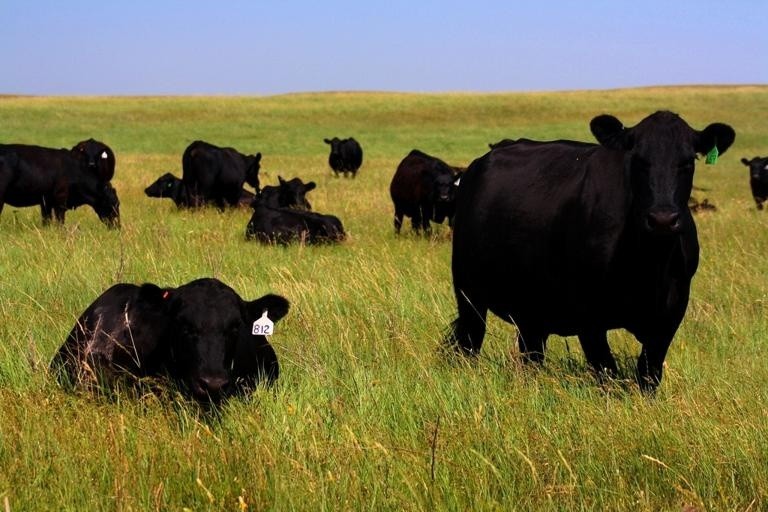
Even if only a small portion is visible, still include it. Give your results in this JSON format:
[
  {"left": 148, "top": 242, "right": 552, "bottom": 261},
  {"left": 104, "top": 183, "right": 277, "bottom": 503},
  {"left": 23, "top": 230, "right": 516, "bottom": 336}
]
[
  {"left": 321, "top": 133, "right": 367, "bottom": 181},
  {"left": 43, "top": 275, "right": 291, "bottom": 425},
  {"left": 386, "top": 144, "right": 464, "bottom": 238},
  {"left": 435, "top": 107, "right": 739, "bottom": 401},
  {"left": 0, "top": 135, "right": 124, "bottom": 230},
  {"left": 740, "top": 153, "right": 768, "bottom": 213},
  {"left": 141, "top": 138, "right": 348, "bottom": 246}
]
